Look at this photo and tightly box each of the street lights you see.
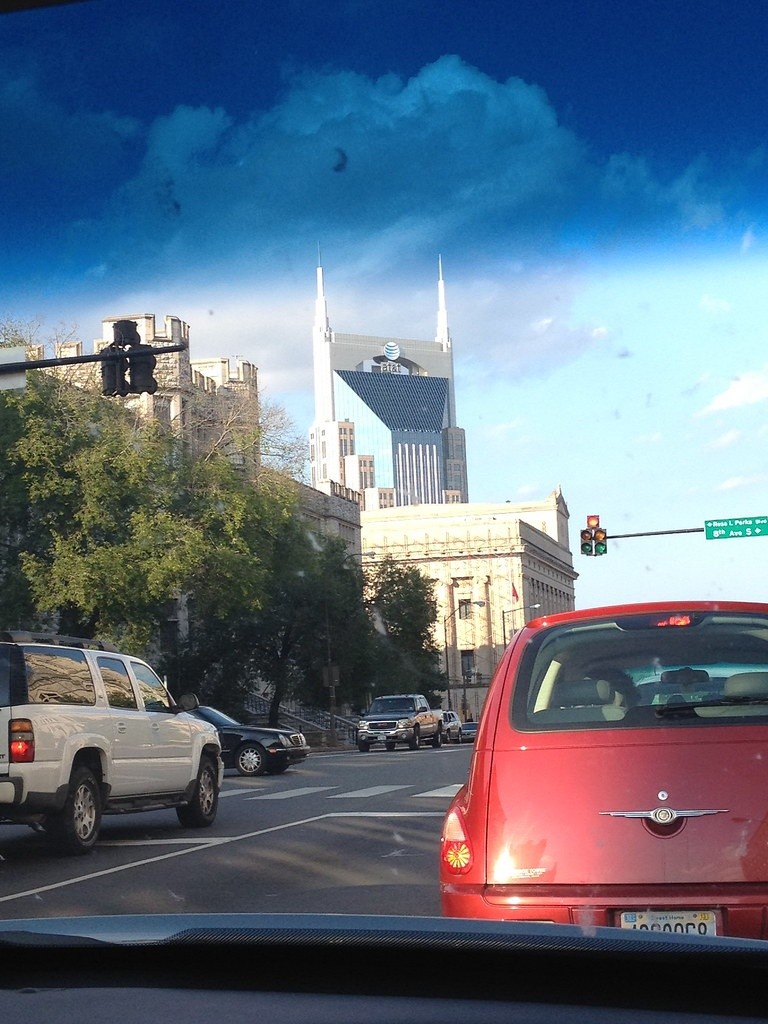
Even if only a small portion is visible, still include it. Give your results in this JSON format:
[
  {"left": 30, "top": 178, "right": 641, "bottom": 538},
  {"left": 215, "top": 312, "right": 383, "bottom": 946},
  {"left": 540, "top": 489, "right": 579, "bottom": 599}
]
[
  {"left": 442, "top": 599, "right": 485, "bottom": 711},
  {"left": 326, "top": 550, "right": 376, "bottom": 749},
  {"left": 502, "top": 603, "right": 541, "bottom": 652}
]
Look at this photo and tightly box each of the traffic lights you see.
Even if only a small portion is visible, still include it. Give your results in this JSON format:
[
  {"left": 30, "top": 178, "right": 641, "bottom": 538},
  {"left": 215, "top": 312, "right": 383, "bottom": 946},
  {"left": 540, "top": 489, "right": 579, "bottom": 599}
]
[
  {"left": 594, "top": 529, "right": 608, "bottom": 556},
  {"left": 580, "top": 529, "right": 593, "bottom": 556},
  {"left": 587, "top": 515, "right": 604, "bottom": 531}
]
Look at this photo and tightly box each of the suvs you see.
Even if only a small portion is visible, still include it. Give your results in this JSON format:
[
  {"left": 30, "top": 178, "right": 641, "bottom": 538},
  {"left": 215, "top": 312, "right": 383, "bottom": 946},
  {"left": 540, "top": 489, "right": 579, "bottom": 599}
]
[
  {"left": 357, "top": 692, "right": 446, "bottom": 750},
  {"left": 0, "top": 630, "right": 225, "bottom": 855}
]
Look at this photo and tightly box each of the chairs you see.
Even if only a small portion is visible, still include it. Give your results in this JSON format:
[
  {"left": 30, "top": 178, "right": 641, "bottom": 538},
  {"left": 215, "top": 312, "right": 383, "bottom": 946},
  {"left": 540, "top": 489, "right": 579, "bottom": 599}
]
[
  {"left": 695, "top": 666, "right": 767, "bottom": 721},
  {"left": 528, "top": 679, "right": 627, "bottom": 726}
]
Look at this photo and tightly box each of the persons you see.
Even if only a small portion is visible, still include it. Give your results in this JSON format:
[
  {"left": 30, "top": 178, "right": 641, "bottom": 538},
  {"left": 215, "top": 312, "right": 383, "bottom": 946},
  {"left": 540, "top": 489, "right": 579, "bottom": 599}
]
[{"left": 592, "top": 665, "right": 641, "bottom": 707}]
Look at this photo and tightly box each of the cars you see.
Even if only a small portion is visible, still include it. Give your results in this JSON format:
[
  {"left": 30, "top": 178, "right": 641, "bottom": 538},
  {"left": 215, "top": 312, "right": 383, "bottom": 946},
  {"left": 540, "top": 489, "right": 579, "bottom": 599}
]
[
  {"left": 461, "top": 722, "right": 481, "bottom": 743},
  {"left": 191, "top": 706, "right": 311, "bottom": 776},
  {"left": 442, "top": 710, "right": 462, "bottom": 743},
  {"left": 437, "top": 603, "right": 768, "bottom": 938}
]
